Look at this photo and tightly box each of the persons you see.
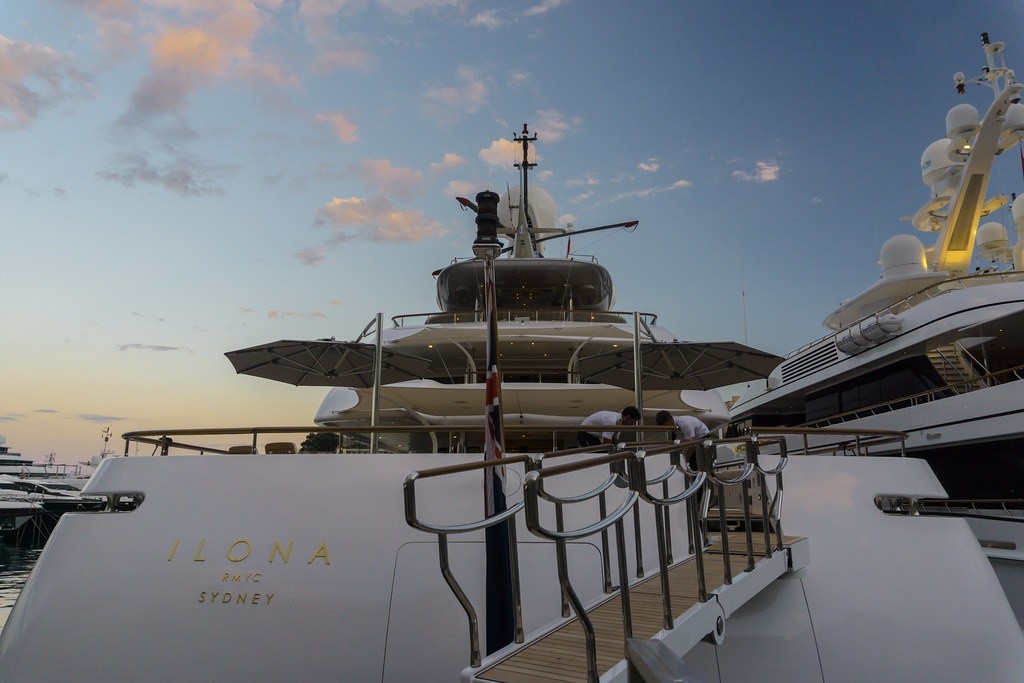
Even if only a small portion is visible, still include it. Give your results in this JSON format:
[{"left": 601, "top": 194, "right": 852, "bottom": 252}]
[
  {"left": 654, "top": 409, "right": 716, "bottom": 503},
  {"left": 575, "top": 406, "right": 642, "bottom": 453}
]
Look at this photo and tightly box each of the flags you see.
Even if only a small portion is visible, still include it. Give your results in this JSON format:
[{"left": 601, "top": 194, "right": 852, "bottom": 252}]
[{"left": 481, "top": 263, "right": 515, "bottom": 655}]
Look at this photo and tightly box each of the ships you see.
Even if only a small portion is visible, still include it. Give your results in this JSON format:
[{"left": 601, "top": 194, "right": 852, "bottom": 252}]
[{"left": 0, "top": 32, "right": 1024, "bottom": 681}]
